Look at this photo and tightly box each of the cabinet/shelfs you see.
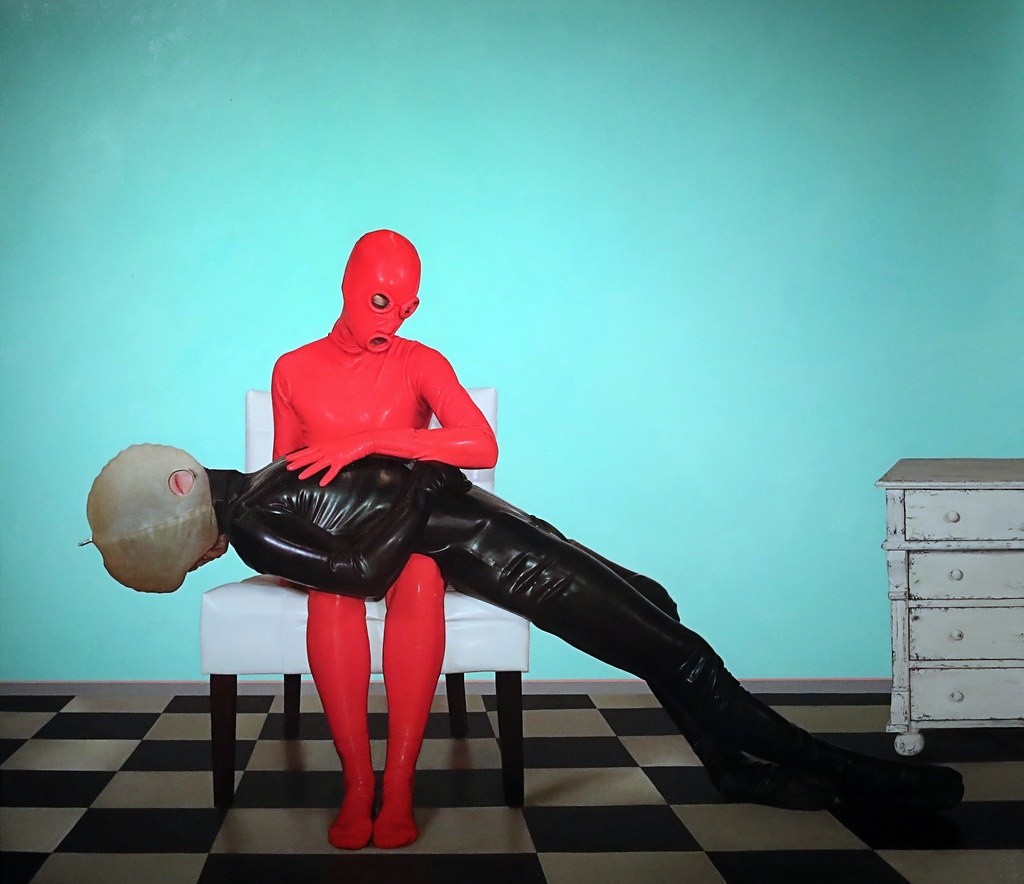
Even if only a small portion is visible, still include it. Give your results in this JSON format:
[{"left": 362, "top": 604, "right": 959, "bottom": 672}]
[{"left": 875, "top": 457, "right": 1024, "bottom": 760}]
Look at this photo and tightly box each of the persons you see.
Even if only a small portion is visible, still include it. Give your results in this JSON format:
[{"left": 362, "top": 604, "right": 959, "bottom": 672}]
[
  {"left": 82, "top": 436, "right": 966, "bottom": 844},
  {"left": 268, "top": 227, "right": 502, "bottom": 846}
]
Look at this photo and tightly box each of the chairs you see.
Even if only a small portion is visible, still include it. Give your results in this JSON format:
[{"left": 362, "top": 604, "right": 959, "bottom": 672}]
[{"left": 191, "top": 385, "right": 533, "bottom": 810}]
